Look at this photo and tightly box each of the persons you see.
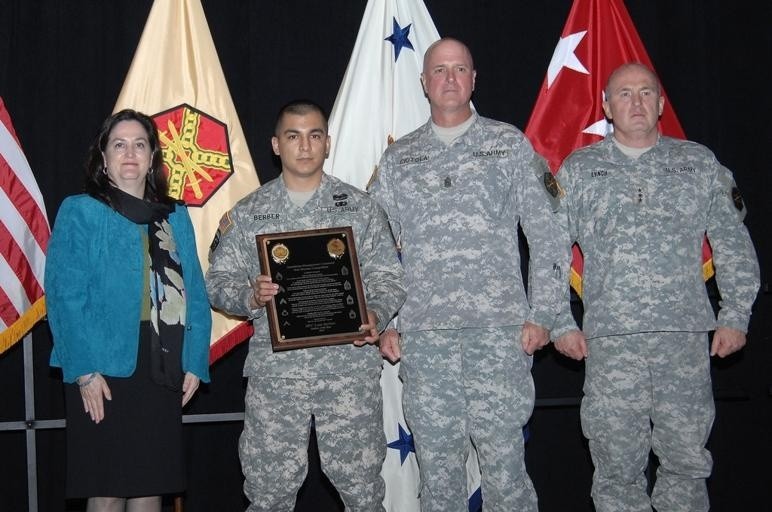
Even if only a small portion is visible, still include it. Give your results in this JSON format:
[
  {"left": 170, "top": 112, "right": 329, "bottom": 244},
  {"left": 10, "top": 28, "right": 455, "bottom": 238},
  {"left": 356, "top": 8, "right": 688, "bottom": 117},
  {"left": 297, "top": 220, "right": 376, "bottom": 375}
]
[
  {"left": 366, "top": 38, "right": 573, "bottom": 511},
  {"left": 550, "top": 62, "right": 762, "bottom": 511},
  {"left": 205, "top": 100, "right": 408, "bottom": 511},
  {"left": 44, "top": 109, "right": 213, "bottom": 512}
]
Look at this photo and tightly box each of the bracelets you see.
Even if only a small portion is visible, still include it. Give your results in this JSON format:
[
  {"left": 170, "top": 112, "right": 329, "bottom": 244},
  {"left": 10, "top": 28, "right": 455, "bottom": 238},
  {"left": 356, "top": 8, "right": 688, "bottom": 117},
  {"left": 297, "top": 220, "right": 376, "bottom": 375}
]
[{"left": 78, "top": 371, "right": 96, "bottom": 387}]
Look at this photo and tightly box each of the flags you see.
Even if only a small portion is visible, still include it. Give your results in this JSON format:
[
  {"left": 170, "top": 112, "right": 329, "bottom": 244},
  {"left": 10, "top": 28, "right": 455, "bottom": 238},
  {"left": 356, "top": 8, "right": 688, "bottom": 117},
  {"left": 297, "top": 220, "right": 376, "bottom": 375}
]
[
  {"left": 1, "top": 97, "right": 53, "bottom": 359},
  {"left": 322, "top": 1, "right": 484, "bottom": 512},
  {"left": 524, "top": 0, "right": 717, "bottom": 304},
  {"left": 110, "top": 0, "right": 262, "bottom": 366}
]
